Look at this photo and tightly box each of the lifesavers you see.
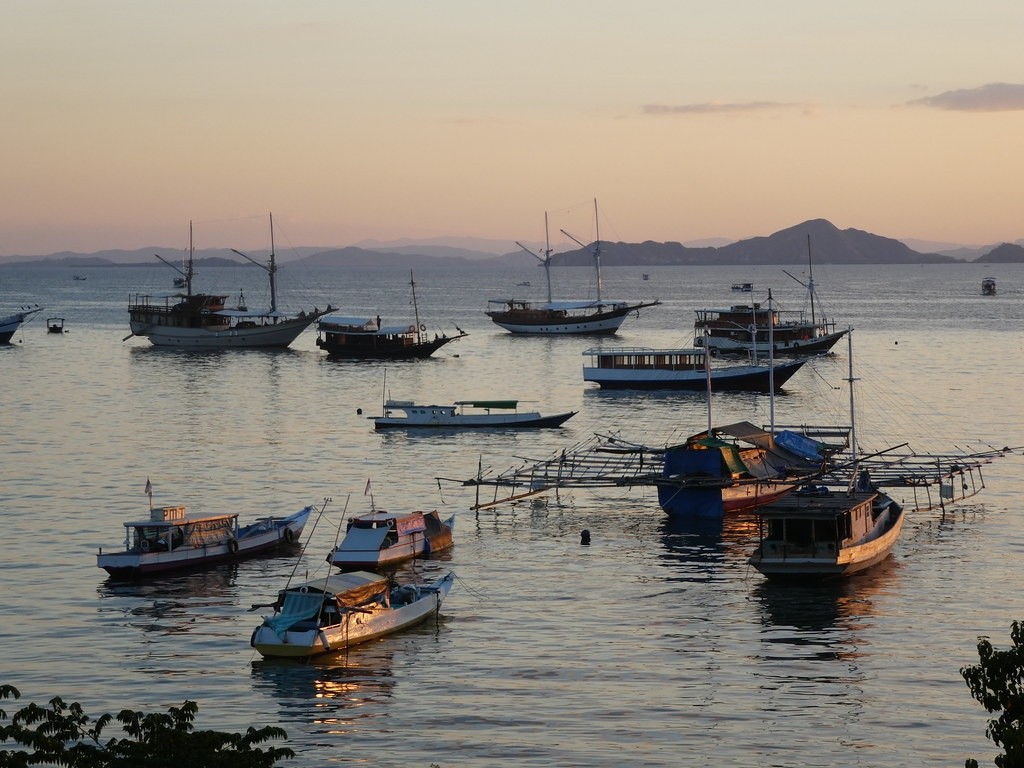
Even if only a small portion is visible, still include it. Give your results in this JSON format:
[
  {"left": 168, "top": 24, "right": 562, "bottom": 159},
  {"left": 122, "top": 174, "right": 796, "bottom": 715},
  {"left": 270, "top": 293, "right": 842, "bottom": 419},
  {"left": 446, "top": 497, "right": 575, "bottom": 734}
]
[
  {"left": 140, "top": 540, "right": 149, "bottom": 550},
  {"left": 284, "top": 528, "right": 294, "bottom": 544},
  {"left": 420, "top": 325, "right": 426, "bottom": 331},
  {"left": 794, "top": 342, "right": 799, "bottom": 348},
  {"left": 409, "top": 326, "right": 415, "bottom": 333},
  {"left": 698, "top": 339, "right": 702, "bottom": 345},
  {"left": 227, "top": 538, "right": 239, "bottom": 554}
]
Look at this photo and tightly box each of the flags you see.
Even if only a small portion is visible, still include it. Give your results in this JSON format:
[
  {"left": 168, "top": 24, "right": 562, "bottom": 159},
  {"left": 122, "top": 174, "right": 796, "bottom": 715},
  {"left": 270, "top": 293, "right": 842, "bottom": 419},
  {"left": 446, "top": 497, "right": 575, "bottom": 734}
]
[
  {"left": 145, "top": 479, "right": 152, "bottom": 497},
  {"left": 364, "top": 479, "right": 371, "bottom": 496}
]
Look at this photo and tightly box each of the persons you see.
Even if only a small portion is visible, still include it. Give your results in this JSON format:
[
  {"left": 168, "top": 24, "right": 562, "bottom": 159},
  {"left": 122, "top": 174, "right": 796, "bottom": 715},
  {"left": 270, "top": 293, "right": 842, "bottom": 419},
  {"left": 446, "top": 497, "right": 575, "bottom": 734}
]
[{"left": 376, "top": 315, "right": 381, "bottom": 331}]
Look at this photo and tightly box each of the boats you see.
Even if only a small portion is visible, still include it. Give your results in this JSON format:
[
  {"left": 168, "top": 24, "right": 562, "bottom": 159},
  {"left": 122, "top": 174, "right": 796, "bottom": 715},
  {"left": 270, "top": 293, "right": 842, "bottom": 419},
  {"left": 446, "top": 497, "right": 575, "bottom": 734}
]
[
  {"left": 642, "top": 273, "right": 650, "bottom": 281},
  {"left": 96, "top": 477, "right": 312, "bottom": 581},
  {"left": 515, "top": 281, "right": 531, "bottom": 286},
  {"left": 123, "top": 211, "right": 339, "bottom": 349},
  {"left": 324, "top": 477, "right": 455, "bottom": 569},
  {"left": 981, "top": 276, "right": 998, "bottom": 297},
  {"left": 485, "top": 197, "right": 664, "bottom": 335},
  {"left": 173, "top": 277, "right": 188, "bottom": 288},
  {"left": 0, "top": 303, "right": 46, "bottom": 344},
  {"left": 73, "top": 275, "right": 88, "bottom": 281},
  {"left": 729, "top": 283, "right": 753, "bottom": 292},
  {"left": 247, "top": 557, "right": 455, "bottom": 659},
  {"left": 365, "top": 367, "right": 579, "bottom": 429}
]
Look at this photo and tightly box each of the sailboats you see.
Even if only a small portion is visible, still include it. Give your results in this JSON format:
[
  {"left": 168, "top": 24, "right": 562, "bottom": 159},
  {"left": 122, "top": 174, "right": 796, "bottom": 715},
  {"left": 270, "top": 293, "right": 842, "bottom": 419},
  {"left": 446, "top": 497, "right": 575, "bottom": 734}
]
[
  {"left": 316, "top": 267, "right": 470, "bottom": 358},
  {"left": 693, "top": 232, "right": 855, "bottom": 354},
  {"left": 582, "top": 322, "right": 807, "bottom": 392},
  {"left": 658, "top": 285, "right": 825, "bottom": 519},
  {"left": 747, "top": 325, "right": 904, "bottom": 574}
]
